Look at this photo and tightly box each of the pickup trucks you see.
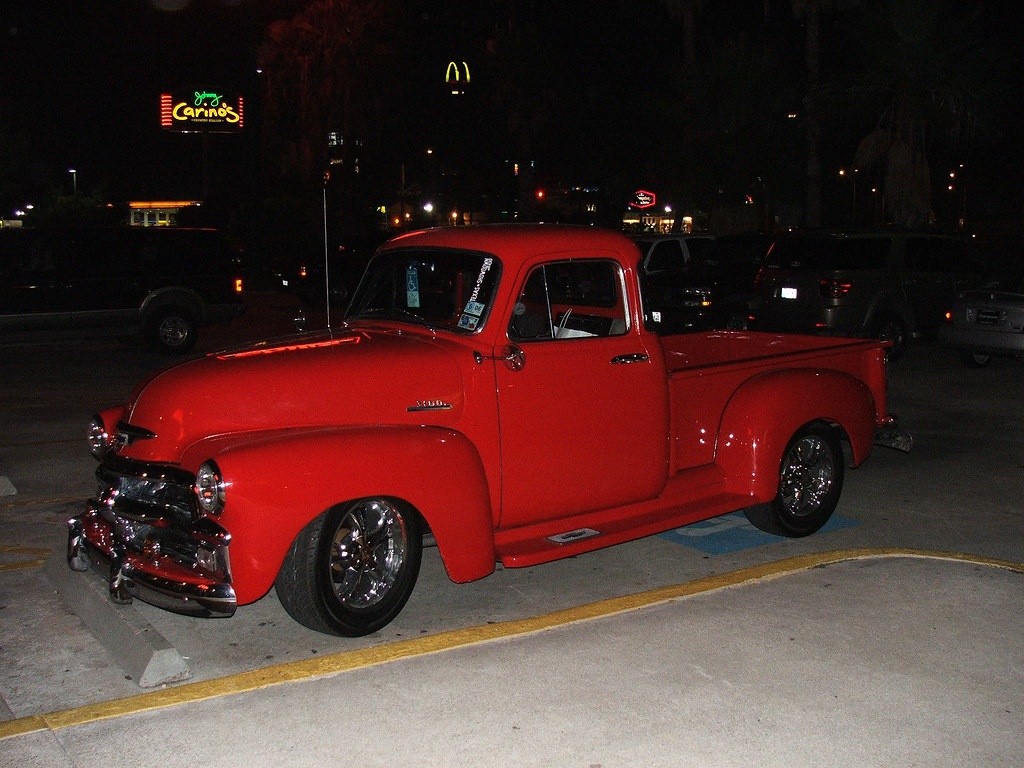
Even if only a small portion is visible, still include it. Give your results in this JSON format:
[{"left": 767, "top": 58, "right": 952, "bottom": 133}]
[{"left": 68, "top": 221, "right": 914, "bottom": 638}]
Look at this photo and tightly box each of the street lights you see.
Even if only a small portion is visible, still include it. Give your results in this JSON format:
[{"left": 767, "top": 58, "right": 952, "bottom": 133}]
[
  {"left": 839, "top": 170, "right": 856, "bottom": 222},
  {"left": 69, "top": 169, "right": 77, "bottom": 198}
]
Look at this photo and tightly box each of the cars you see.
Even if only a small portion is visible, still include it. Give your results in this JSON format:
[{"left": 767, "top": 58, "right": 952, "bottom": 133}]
[
  {"left": 630, "top": 234, "right": 1023, "bottom": 370},
  {"left": 0, "top": 205, "right": 403, "bottom": 359}
]
